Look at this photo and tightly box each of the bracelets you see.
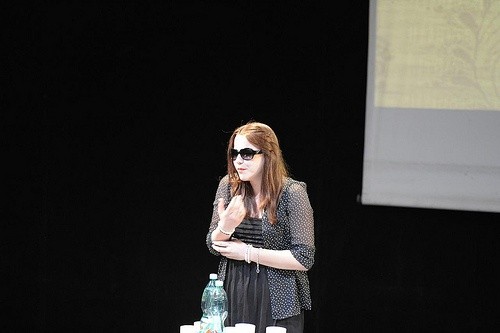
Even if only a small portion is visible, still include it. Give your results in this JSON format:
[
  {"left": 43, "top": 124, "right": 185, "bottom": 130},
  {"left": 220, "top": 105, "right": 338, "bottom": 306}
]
[
  {"left": 218, "top": 222, "right": 235, "bottom": 236},
  {"left": 256, "top": 247, "right": 262, "bottom": 273},
  {"left": 245, "top": 244, "right": 253, "bottom": 264}
]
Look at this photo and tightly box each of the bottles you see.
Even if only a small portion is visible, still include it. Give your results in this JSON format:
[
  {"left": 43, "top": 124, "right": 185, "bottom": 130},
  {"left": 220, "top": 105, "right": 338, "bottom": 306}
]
[
  {"left": 206, "top": 281, "right": 229, "bottom": 333},
  {"left": 199, "top": 273, "right": 217, "bottom": 333}
]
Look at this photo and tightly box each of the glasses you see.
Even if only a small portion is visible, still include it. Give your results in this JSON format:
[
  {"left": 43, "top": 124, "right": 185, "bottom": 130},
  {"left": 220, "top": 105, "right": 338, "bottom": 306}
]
[{"left": 229, "top": 148, "right": 263, "bottom": 160}]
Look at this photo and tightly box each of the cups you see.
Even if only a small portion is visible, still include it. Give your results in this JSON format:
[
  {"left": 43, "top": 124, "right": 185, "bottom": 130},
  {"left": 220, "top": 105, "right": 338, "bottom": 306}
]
[
  {"left": 266, "top": 326, "right": 286, "bottom": 333},
  {"left": 194, "top": 322, "right": 201, "bottom": 333},
  {"left": 180, "top": 326, "right": 195, "bottom": 333},
  {"left": 224, "top": 327, "right": 235, "bottom": 333},
  {"left": 235, "top": 323, "right": 255, "bottom": 333}
]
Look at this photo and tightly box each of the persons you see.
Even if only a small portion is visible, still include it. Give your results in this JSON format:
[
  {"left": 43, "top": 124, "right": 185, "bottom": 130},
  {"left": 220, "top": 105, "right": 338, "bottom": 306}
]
[{"left": 206, "top": 123, "right": 315, "bottom": 333}]
